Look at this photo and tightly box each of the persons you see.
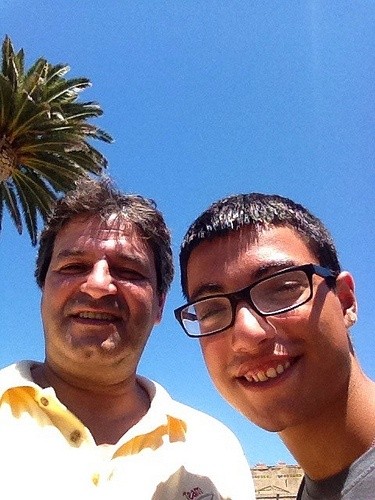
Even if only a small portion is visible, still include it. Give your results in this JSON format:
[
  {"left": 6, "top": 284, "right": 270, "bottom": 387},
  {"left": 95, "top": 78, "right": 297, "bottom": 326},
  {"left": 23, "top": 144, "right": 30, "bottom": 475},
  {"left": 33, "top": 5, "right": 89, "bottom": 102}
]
[
  {"left": 1, "top": 180, "right": 255, "bottom": 500},
  {"left": 178, "top": 190, "right": 375, "bottom": 500}
]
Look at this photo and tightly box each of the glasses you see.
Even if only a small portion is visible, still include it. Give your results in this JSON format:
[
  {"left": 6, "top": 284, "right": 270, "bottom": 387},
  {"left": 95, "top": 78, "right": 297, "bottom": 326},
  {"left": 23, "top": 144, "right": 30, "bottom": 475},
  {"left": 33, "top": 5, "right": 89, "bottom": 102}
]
[{"left": 174, "top": 263, "right": 340, "bottom": 338}]
